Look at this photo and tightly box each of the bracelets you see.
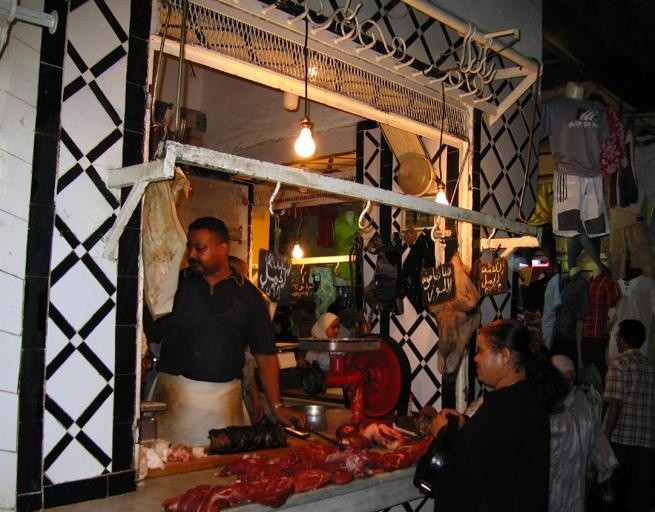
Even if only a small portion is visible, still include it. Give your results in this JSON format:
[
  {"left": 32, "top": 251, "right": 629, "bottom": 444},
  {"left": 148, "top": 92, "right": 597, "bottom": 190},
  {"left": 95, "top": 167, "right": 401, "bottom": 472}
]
[{"left": 271, "top": 401, "right": 285, "bottom": 411}]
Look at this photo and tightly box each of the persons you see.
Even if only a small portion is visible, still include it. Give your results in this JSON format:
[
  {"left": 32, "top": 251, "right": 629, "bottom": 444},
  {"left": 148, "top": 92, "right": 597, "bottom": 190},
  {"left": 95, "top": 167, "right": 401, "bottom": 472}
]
[
  {"left": 540, "top": 79, "right": 610, "bottom": 238},
  {"left": 331, "top": 210, "right": 360, "bottom": 282},
  {"left": 541, "top": 265, "right": 654, "bottom": 370},
  {"left": 227, "top": 254, "right": 271, "bottom": 423},
  {"left": 142, "top": 216, "right": 309, "bottom": 448},
  {"left": 273, "top": 294, "right": 358, "bottom": 369}
]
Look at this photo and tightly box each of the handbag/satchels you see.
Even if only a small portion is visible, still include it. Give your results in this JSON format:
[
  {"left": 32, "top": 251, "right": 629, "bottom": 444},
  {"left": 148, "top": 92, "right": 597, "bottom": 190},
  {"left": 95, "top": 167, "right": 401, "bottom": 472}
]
[{"left": 412, "top": 414, "right": 461, "bottom": 499}]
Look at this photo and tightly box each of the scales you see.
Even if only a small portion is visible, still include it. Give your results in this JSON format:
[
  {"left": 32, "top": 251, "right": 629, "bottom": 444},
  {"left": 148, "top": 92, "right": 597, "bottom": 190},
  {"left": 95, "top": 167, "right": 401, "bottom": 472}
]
[{"left": 273, "top": 343, "right": 297, "bottom": 369}]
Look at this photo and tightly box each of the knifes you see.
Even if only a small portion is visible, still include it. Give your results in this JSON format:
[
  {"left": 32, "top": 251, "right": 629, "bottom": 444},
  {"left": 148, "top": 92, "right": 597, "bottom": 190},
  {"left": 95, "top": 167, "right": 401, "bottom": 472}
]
[{"left": 283, "top": 426, "right": 315, "bottom": 442}]
